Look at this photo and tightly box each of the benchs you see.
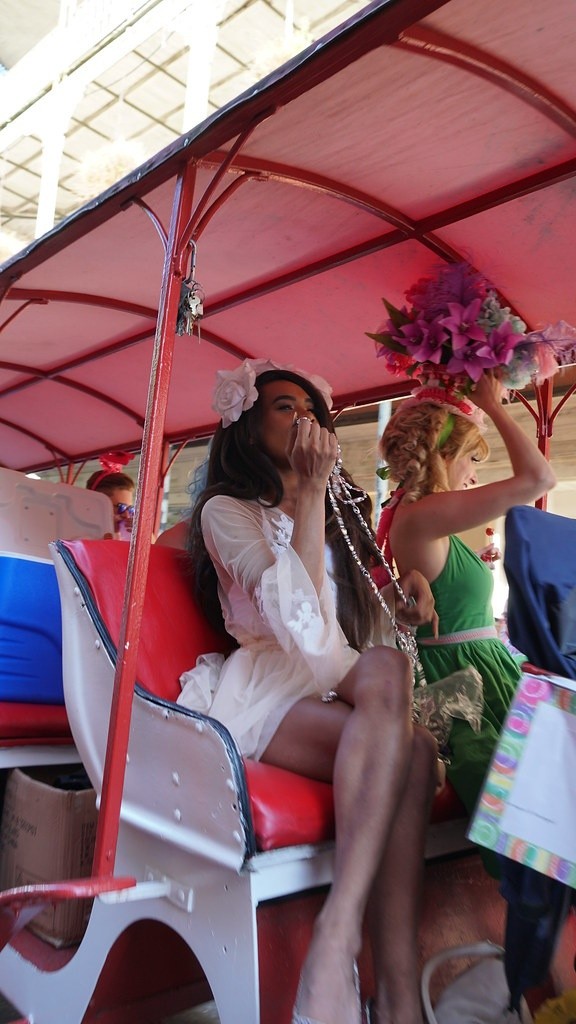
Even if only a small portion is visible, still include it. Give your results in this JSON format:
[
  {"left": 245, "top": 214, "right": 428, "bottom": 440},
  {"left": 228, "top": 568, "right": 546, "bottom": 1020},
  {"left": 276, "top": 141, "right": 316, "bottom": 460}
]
[
  {"left": 40, "top": 534, "right": 484, "bottom": 902},
  {"left": 1, "top": 464, "right": 113, "bottom": 770}
]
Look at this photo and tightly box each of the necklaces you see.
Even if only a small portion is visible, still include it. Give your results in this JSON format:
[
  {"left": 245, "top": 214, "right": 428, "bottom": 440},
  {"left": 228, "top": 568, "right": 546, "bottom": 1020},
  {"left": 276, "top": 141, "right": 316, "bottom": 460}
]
[{"left": 297, "top": 417, "right": 427, "bottom": 688}]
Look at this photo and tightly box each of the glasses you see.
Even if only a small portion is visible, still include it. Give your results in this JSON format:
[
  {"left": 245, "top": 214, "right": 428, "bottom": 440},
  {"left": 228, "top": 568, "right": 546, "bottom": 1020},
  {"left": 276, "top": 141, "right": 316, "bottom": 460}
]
[{"left": 114, "top": 503, "right": 135, "bottom": 516}]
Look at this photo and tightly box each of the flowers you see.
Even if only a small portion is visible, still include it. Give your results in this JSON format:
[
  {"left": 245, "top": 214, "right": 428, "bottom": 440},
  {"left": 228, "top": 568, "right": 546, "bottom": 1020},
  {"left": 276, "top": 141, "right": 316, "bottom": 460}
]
[
  {"left": 210, "top": 361, "right": 336, "bottom": 431},
  {"left": 372, "top": 258, "right": 576, "bottom": 399}
]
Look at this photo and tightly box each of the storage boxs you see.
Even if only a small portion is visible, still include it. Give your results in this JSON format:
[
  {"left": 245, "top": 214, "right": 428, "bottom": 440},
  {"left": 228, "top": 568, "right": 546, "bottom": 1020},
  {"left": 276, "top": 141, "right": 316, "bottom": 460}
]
[{"left": 0, "top": 766, "right": 100, "bottom": 949}]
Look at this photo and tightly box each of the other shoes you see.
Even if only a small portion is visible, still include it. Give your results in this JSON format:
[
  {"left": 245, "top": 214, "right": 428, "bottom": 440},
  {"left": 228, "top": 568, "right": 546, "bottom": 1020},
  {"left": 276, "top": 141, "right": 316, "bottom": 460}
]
[
  {"left": 291, "top": 954, "right": 362, "bottom": 1024},
  {"left": 367, "top": 990, "right": 425, "bottom": 1024}
]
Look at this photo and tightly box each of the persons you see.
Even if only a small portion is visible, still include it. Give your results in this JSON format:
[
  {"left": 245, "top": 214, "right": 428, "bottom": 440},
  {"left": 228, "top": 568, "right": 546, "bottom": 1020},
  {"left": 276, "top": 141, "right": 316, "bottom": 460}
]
[
  {"left": 86, "top": 470, "right": 136, "bottom": 533},
  {"left": 379, "top": 371, "right": 576, "bottom": 1024},
  {"left": 177, "top": 360, "right": 440, "bottom": 1023}
]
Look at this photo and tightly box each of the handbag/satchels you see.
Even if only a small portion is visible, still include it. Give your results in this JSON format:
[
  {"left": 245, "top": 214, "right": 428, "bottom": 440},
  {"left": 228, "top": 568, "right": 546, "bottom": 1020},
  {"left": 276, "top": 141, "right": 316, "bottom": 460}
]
[{"left": 422, "top": 943, "right": 534, "bottom": 1024}]
